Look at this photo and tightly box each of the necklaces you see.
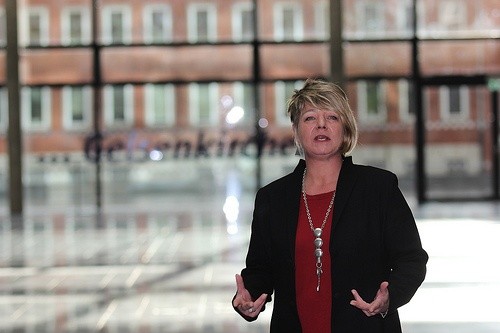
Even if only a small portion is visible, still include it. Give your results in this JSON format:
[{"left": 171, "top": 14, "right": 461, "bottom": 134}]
[{"left": 302, "top": 162, "right": 336, "bottom": 292}]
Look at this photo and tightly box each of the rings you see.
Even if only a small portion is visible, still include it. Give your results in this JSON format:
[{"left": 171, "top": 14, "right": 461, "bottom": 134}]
[{"left": 248, "top": 308, "right": 256, "bottom": 312}]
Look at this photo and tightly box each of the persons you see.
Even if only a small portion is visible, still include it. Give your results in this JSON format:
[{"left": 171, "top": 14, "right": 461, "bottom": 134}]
[{"left": 230, "top": 78, "right": 428, "bottom": 333}]
